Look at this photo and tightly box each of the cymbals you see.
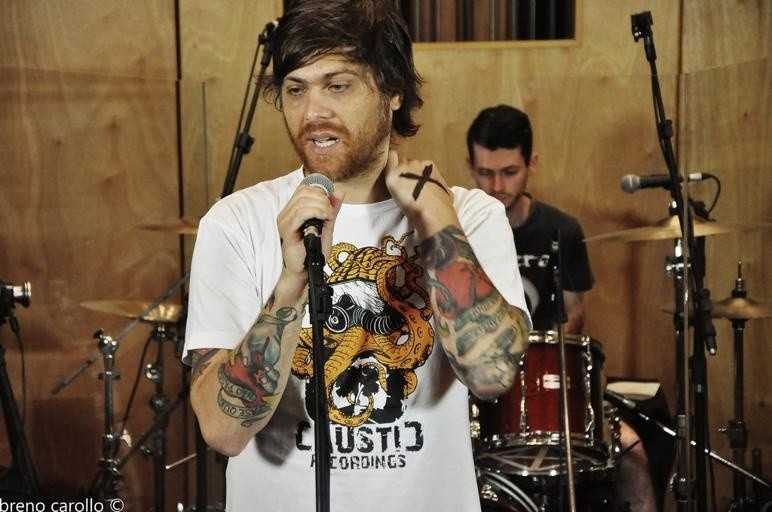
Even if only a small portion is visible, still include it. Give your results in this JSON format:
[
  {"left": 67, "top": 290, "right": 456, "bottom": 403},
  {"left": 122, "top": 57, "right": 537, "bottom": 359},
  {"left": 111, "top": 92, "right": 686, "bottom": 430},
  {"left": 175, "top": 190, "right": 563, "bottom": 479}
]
[
  {"left": 661, "top": 297, "right": 771, "bottom": 320},
  {"left": 80, "top": 300, "right": 184, "bottom": 324},
  {"left": 581, "top": 212, "right": 731, "bottom": 244},
  {"left": 135, "top": 222, "right": 199, "bottom": 236}
]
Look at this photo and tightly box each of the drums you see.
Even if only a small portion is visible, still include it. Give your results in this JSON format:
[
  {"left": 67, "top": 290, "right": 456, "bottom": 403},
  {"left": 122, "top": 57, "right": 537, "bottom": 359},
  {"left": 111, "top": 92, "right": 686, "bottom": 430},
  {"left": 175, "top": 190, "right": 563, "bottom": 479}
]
[{"left": 468, "top": 330, "right": 611, "bottom": 477}]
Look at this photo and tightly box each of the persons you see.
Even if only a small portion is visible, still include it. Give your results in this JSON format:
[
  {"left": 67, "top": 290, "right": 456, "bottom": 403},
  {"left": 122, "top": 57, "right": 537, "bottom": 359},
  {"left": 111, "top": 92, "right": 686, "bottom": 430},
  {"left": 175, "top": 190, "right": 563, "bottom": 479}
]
[
  {"left": 181, "top": 2, "right": 536, "bottom": 510},
  {"left": 463, "top": 102, "right": 658, "bottom": 510}
]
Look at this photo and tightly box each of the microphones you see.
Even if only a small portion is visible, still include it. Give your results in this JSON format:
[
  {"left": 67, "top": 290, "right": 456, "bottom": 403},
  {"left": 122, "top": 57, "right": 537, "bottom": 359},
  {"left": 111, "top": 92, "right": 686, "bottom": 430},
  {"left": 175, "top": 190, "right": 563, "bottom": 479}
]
[
  {"left": 299, "top": 172, "right": 335, "bottom": 251},
  {"left": 620, "top": 173, "right": 710, "bottom": 193}
]
